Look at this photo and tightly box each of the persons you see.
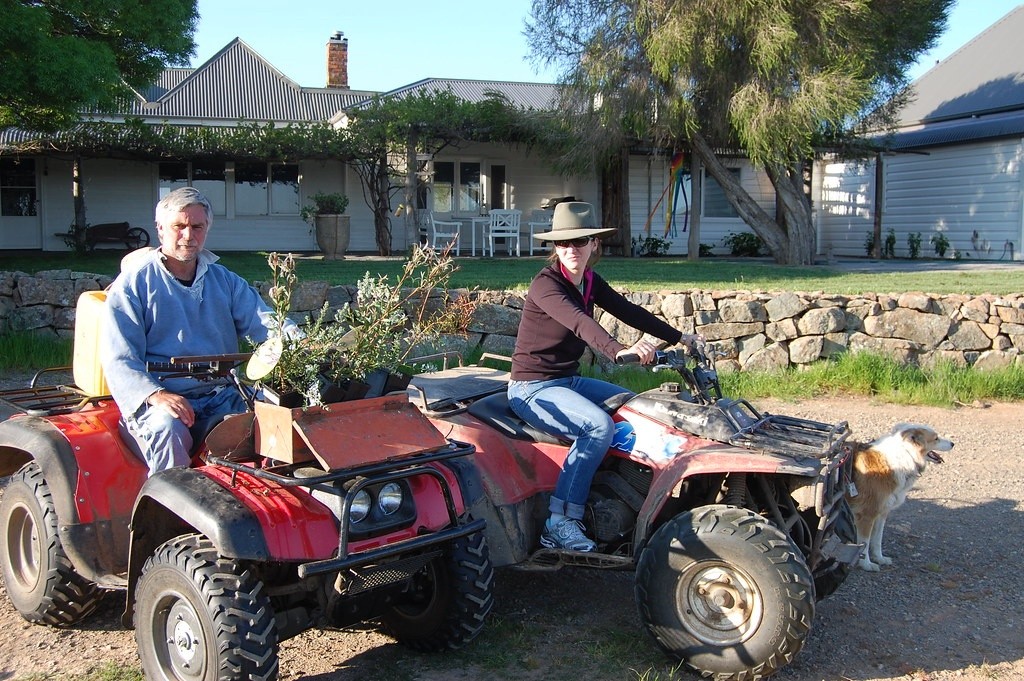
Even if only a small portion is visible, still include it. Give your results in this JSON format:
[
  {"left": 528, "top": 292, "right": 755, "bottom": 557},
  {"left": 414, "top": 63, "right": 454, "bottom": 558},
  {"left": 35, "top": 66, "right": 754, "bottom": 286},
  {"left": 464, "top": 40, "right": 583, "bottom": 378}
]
[
  {"left": 99, "top": 187, "right": 307, "bottom": 477},
  {"left": 507, "top": 202, "right": 705, "bottom": 559}
]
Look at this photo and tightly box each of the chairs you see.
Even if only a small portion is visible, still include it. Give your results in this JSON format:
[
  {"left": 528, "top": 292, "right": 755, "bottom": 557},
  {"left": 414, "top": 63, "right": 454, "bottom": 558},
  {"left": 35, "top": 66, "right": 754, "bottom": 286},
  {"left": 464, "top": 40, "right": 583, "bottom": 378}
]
[{"left": 482, "top": 209, "right": 522, "bottom": 258}]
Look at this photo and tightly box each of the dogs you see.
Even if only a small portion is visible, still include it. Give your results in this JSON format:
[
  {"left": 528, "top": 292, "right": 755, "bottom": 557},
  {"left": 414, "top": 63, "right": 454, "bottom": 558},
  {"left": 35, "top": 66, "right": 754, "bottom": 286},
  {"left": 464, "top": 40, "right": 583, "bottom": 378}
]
[{"left": 833, "top": 422, "right": 955, "bottom": 572}]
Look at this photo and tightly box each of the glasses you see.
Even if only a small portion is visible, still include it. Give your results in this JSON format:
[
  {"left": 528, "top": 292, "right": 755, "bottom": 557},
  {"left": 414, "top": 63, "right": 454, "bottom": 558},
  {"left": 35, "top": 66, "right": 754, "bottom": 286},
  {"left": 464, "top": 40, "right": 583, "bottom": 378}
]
[{"left": 552, "top": 236, "right": 594, "bottom": 248}]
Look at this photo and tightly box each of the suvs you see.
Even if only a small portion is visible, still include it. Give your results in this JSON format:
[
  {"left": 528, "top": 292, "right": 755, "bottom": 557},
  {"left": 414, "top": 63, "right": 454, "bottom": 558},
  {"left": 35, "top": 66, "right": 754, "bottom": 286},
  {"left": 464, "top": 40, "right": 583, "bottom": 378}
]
[
  {"left": 390, "top": 340, "right": 868, "bottom": 680},
  {"left": 0, "top": 288, "right": 497, "bottom": 681}
]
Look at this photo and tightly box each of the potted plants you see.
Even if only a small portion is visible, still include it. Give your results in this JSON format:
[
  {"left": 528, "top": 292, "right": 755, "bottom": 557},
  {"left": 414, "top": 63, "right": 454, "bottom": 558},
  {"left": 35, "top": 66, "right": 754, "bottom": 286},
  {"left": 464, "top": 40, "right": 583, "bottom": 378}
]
[
  {"left": 885, "top": 229, "right": 896, "bottom": 259},
  {"left": 296, "top": 189, "right": 352, "bottom": 260},
  {"left": 864, "top": 231, "right": 882, "bottom": 258}
]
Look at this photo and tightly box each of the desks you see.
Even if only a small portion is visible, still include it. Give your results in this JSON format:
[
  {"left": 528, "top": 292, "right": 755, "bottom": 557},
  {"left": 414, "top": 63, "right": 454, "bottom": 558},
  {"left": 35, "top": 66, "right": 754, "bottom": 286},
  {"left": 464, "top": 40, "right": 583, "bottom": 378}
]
[{"left": 446, "top": 217, "right": 520, "bottom": 256}]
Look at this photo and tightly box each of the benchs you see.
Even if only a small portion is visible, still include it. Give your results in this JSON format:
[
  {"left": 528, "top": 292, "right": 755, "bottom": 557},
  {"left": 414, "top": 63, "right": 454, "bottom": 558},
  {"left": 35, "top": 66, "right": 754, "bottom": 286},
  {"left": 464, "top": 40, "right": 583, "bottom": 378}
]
[
  {"left": 510, "top": 208, "right": 555, "bottom": 256},
  {"left": 414, "top": 209, "right": 463, "bottom": 257}
]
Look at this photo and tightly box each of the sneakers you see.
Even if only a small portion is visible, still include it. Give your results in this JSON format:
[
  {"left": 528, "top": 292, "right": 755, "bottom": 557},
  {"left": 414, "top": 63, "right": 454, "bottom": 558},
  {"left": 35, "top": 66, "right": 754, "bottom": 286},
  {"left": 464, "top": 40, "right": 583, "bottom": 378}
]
[{"left": 540, "top": 518, "right": 597, "bottom": 552}]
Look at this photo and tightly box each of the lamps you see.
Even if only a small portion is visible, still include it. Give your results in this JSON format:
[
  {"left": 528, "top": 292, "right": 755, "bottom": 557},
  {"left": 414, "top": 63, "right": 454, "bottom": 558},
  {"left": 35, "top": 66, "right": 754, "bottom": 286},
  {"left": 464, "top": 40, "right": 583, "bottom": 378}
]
[{"left": 479, "top": 204, "right": 489, "bottom": 218}]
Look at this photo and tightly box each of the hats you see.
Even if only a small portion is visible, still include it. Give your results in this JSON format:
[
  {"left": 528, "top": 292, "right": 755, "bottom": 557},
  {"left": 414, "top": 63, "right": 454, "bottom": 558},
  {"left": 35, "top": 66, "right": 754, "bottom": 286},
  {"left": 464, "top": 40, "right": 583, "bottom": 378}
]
[{"left": 532, "top": 203, "right": 618, "bottom": 240}]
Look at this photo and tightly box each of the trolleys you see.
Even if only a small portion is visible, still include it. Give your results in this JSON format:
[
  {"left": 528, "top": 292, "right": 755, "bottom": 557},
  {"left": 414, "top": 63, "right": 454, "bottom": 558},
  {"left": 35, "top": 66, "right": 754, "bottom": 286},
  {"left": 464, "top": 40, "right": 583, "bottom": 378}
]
[{"left": 54, "top": 220, "right": 150, "bottom": 251}]
